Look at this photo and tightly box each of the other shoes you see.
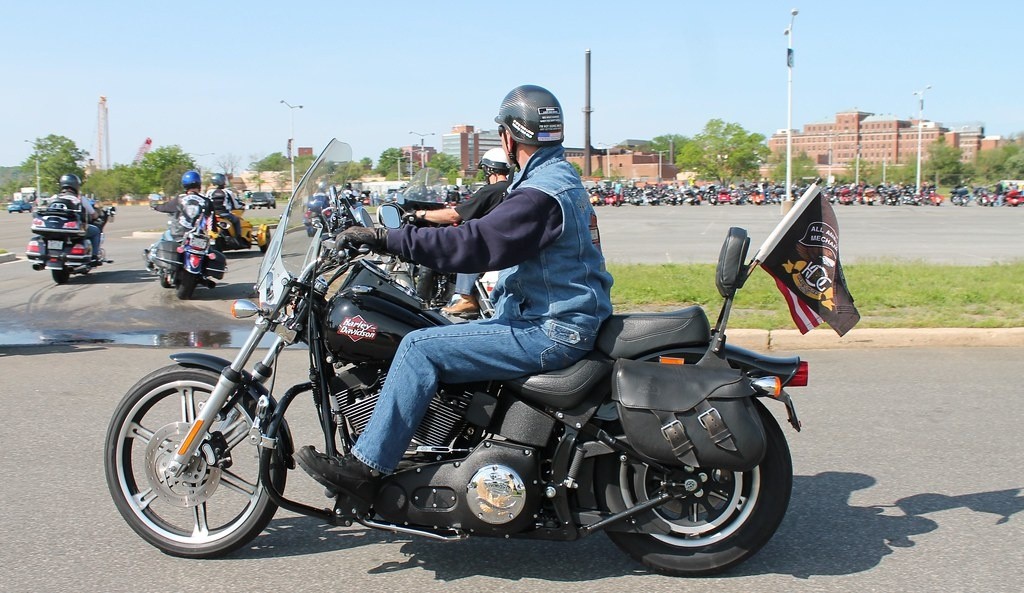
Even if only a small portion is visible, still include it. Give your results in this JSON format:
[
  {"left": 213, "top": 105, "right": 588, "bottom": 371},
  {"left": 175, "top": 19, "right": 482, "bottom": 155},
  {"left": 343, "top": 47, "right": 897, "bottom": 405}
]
[
  {"left": 90, "top": 257, "right": 102, "bottom": 267},
  {"left": 145, "top": 248, "right": 154, "bottom": 268},
  {"left": 441, "top": 295, "right": 476, "bottom": 315}
]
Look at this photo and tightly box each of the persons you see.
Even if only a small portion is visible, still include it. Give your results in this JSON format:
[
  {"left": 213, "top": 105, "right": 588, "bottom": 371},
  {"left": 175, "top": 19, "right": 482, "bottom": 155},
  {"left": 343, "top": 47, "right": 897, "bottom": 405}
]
[
  {"left": 589, "top": 181, "right": 604, "bottom": 204},
  {"left": 754, "top": 184, "right": 861, "bottom": 337},
  {"left": 461, "top": 184, "right": 473, "bottom": 195},
  {"left": 343, "top": 183, "right": 360, "bottom": 202},
  {"left": 855, "top": 178, "right": 869, "bottom": 190},
  {"left": 680, "top": 183, "right": 686, "bottom": 189},
  {"left": 313, "top": 182, "right": 330, "bottom": 208},
  {"left": 47, "top": 174, "right": 103, "bottom": 266},
  {"left": 400, "top": 147, "right": 511, "bottom": 313},
  {"left": 448, "top": 185, "right": 460, "bottom": 202},
  {"left": 206, "top": 172, "right": 245, "bottom": 244},
  {"left": 739, "top": 180, "right": 746, "bottom": 188},
  {"left": 292, "top": 84, "right": 614, "bottom": 502},
  {"left": 991, "top": 180, "right": 1002, "bottom": 195},
  {"left": 775, "top": 179, "right": 784, "bottom": 190},
  {"left": 644, "top": 182, "right": 652, "bottom": 189},
  {"left": 373, "top": 190, "right": 379, "bottom": 206},
  {"left": 614, "top": 179, "right": 624, "bottom": 207},
  {"left": 730, "top": 181, "right": 737, "bottom": 190},
  {"left": 668, "top": 182, "right": 674, "bottom": 189},
  {"left": 603, "top": 183, "right": 610, "bottom": 188},
  {"left": 145, "top": 171, "right": 217, "bottom": 267}
]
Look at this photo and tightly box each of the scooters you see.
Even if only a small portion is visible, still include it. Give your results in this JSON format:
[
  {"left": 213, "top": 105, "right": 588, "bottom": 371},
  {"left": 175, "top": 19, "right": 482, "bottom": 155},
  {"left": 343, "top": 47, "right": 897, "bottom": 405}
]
[{"left": 301, "top": 179, "right": 1024, "bottom": 321}]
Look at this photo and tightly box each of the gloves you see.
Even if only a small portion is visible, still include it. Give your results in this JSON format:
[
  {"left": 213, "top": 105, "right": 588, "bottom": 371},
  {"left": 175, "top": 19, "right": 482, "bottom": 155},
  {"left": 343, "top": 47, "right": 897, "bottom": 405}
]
[
  {"left": 150, "top": 200, "right": 158, "bottom": 209},
  {"left": 335, "top": 224, "right": 388, "bottom": 254}
]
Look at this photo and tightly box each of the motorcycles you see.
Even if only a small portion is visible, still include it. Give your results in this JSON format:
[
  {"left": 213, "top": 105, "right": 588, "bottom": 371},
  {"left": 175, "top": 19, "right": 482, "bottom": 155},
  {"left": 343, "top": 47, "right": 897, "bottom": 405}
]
[
  {"left": 25, "top": 199, "right": 117, "bottom": 285},
  {"left": 139, "top": 194, "right": 229, "bottom": 300},
  {"left": 204, "top": 198, "right": 271, "bottom": 253},
  {"left": 103, "top": 138, "right": 809, "bottom": 578}
]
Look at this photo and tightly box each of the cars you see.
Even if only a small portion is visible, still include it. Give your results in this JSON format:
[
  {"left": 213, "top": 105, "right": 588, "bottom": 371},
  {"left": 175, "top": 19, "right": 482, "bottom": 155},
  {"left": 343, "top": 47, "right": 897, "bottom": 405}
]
[
  {"left": 248, "top": 191, "right": 276, "bottom": 209},
  {"left": 7, "top": 200, "right": 33, "bottom": 213}
]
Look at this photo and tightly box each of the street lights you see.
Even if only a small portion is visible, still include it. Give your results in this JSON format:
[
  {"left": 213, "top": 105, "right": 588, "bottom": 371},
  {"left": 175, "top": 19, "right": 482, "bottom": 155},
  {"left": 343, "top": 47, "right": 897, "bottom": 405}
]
[
  {"left": 280, "top": 99, "right": 304, "bottom": 203},
  {"left": 782, "top": 7, "right": 799, "bottom": 220},
  {"left": 408, "top": 131, "right": 436, "bottom": 169},
  {"left": 913, "top": 85, "right": 931, "bottom": 193},
  {"left": 386, "top": 149, "right": 418, "bottom": 181},
  {"left": 24, "top": 140, "right": 43, "bottom": 199}
]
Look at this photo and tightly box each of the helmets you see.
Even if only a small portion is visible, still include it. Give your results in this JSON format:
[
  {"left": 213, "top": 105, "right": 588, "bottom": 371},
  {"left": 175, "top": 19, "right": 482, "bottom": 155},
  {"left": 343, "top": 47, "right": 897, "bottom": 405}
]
[
  {"left": 59, "top": 173, "right": 80, "bottom": 193},
  {"left": 318, "top": 182, "right": 326, "bottom": 191},
  {"left": 211, "top": 172, "right": 226, "bottom": 186},
  {"left": 344, "top": 182, "right": 351, "bottom": 189},
  {"left": 494, "top": 85, "right": 564, "bottom": 146},
  {"left": 477, "top": 147, "right": 511, "bottom": 174},
  {"left": 182, "top": 171, "right": 201, "bottom": 187}
]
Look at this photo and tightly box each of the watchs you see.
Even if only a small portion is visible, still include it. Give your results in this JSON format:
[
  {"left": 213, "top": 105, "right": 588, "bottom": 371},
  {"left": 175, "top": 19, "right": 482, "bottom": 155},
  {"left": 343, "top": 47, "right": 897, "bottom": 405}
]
[{"left": 421, "top": 209, "right": 426, "bottom": 219}]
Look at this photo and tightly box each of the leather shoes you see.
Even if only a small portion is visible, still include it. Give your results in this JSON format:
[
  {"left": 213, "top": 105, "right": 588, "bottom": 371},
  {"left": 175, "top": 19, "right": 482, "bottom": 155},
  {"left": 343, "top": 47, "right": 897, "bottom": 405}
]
[{"left": 293, "top": 444, "right": 382, "bottom": 512}]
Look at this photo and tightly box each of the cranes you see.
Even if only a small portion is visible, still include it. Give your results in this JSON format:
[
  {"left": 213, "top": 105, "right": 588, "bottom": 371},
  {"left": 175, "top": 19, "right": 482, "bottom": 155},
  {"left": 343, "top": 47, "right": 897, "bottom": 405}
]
[{"left": 130, "top": 136, "right": 152, "bottom": 164}]
